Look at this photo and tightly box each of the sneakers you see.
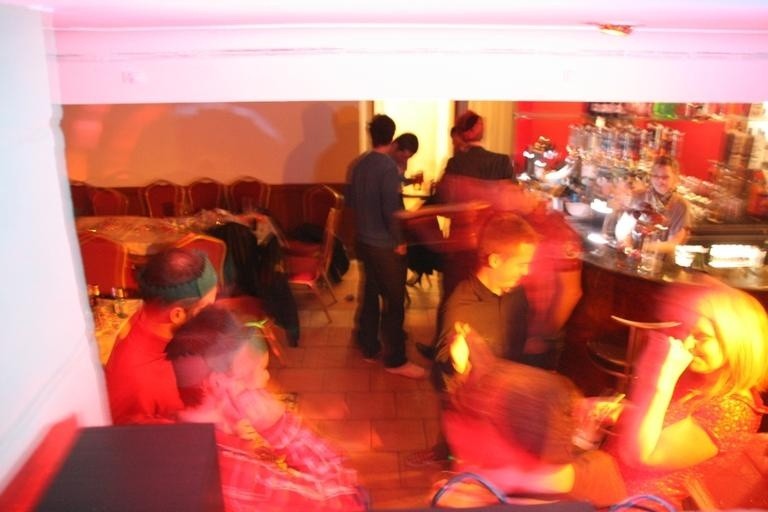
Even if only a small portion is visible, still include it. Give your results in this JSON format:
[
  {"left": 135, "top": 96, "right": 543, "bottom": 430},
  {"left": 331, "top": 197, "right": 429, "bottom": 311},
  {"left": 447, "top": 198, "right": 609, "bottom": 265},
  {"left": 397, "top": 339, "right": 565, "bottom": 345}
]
[
  {"left": 385, "top": 359, "right": 425, "bottom": 380},
  {"left": 406, "top": 447, "right": 451, "bottom": 467}
]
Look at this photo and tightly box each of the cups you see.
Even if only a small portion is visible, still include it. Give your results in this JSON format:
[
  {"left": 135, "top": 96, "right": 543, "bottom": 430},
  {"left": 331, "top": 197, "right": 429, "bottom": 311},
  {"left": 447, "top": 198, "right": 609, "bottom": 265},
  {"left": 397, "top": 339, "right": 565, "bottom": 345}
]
[
  {"left": 571, "top": 393, "right": 624, "bottom": 452},
  {"left": 215, "top": 215, "right": 225, "bottom": 225},
  {"left": 412, "top": 170, "right": 423, "bottom": 191}
]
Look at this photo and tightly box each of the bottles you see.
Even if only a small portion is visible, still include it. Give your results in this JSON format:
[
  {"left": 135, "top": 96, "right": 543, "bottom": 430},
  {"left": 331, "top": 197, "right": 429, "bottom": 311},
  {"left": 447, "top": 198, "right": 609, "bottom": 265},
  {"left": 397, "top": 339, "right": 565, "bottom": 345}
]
[
  {"left": 508, "top": 101, "right": 768, "bottom": 275},
  {"left": 251, "top": 218, "right": 256, "bottom": 230},
  {"left": 86, "top": 284, "right": 130, "bottom": 331}
]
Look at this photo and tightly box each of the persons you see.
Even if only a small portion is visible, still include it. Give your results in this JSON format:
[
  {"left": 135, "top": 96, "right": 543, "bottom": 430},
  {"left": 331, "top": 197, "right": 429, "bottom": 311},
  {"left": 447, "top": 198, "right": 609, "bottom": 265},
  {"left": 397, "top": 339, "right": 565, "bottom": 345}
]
[{"left": 100, "top": 111, "right": 768, "bottom": 512}]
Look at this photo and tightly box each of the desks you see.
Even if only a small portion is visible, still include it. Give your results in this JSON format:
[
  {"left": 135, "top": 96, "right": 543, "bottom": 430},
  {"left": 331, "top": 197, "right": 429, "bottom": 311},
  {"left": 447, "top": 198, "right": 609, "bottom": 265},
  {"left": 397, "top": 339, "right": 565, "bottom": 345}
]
[{"left": 73, "top": 216, "right": 273, "bottom": 268}]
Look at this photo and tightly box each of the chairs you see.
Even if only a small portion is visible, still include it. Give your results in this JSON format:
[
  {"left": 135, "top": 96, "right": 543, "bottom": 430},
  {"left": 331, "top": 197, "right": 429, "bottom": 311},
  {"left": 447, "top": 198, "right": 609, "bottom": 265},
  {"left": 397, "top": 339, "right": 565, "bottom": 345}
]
[
  {"left": 276, "top": 184, "right": 345, "bottom": 323},
  {"left": 74, "top": 230, "right": 131, "bottom": 326},
  {"left": 141, "top": 181, "right": 185, "bottom": 219},
  {"left": 88, "top": 187, "right": 132, "bottom": 215},
  {"left": 70, "top": 179, "right": 92, "bottom": 215},
  {"left": 179, "top": 234, "right": 229, "bottom": 295},
  {"left": 188, "top": 174, "right": 223, "bottom": 213},
  {"left": 587, "top": 310, "right": 687, "bottom": 401},
  {"left": 228, "top": 176, "right": 270, "bottom": 212}
]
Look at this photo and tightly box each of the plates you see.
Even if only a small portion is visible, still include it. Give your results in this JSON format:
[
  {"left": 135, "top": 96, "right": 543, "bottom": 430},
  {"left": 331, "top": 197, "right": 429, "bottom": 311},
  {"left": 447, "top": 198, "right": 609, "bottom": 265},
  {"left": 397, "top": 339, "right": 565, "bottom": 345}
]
[{"left": 86, "top": 217, "right": 177, "bottom": 241}]
